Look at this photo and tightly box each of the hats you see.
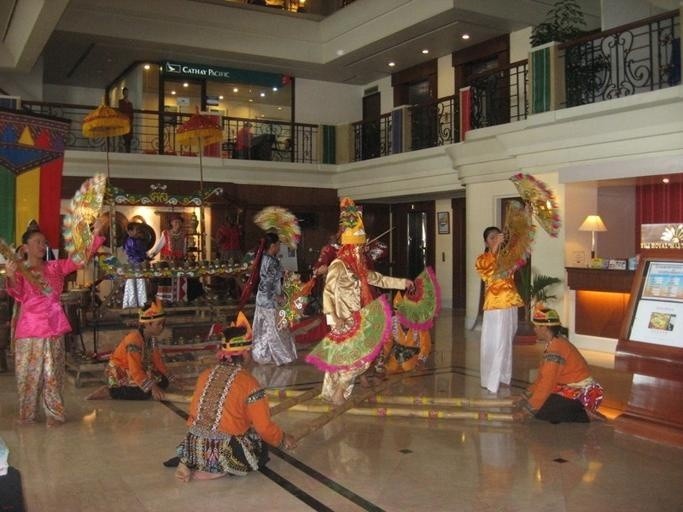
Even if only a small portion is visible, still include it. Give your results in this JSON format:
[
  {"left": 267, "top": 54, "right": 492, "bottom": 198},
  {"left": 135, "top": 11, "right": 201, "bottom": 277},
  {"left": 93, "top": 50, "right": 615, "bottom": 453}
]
[
  {"left": 531, "top": 308, "right": 560, "bottom": 327},
  {"left": 220, "top": 310, "right": 254, "bottom": 353},
  {"left": 138, "top": 294, "right": 166, "bottom": 323},
  {"left": 338, "top": 196, "right": 368, "bottom": 246}
]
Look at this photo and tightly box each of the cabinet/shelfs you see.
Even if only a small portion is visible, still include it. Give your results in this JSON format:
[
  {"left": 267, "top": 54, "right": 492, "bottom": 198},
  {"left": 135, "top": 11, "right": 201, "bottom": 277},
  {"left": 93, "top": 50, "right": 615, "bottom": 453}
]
[{"left": 565, "top": 265, "right": 636, "bottom": 354}]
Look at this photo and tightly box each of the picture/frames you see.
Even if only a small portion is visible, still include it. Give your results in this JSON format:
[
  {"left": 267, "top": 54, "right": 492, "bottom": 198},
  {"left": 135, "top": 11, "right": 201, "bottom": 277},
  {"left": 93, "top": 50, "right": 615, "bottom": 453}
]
[{"left": 437, "top": 211, "right": 450, "bottom": 234}]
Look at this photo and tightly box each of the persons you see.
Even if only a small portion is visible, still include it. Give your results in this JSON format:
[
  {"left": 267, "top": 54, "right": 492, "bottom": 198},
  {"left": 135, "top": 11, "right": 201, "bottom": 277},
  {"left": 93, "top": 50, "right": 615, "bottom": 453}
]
[
  {"left": 233, "top": 124, "right": 252, "bottom": 149},
  {"left": 312, "top": 197, "right": 387, "bottom": 275},
  {"left": 147, "top": 214, "right": 189, "bottom": 306},
  {"left": 5, "top": 217, "right": 108, "bottom": 427},
  {"left": 163, "top": 312, "right": 297, "bottom": 482},
  {"left": 122, "top": 222, "right": 149, "bottom": 308},
  {"left": 318, "top": 205, "right": 416, "bottom": 406},
  {"left": 216, "top": 214, "right": 242, "bottom": 264},
  {"left": 85, "top": 295, "right": 187, "bottom": 401},
  {"left": 375, "top": 310, "right": 431, "bottom": 385},
  {"left": 503, "top": 306, "right": 603, "bottom": 424},
  {"left": 251, "top": 231, "right": 298, "bottom": 366},
  {"left": 118, "top": 87, "right": 134, "bottom": 152},
  {"left": 474, "top": 226, "right": 524, "bottom": 393}
]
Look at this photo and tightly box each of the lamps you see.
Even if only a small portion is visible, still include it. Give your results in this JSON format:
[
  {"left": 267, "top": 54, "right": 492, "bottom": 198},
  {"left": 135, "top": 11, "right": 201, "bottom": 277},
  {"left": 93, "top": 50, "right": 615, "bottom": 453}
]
[{"left": 577, "top": 214, "right": 607, "bottom": 259}]
